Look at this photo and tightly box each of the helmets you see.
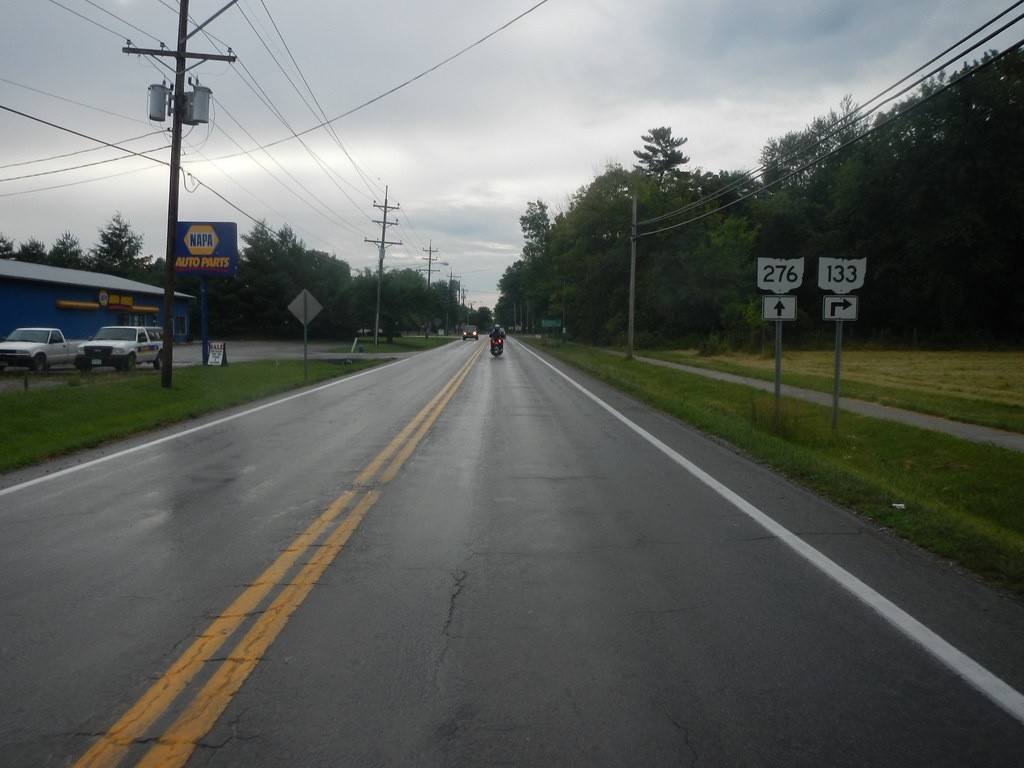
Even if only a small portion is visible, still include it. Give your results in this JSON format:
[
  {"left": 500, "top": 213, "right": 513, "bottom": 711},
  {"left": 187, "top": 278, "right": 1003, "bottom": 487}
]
[{"left": 495, "top": 324, "right": 500, "bottom": 329}]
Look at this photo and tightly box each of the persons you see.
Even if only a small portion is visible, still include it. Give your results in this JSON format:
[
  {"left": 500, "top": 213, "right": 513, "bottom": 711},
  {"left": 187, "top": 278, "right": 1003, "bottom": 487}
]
[{"left": 490, "top": 324, "right": 504, "bottom": 353}]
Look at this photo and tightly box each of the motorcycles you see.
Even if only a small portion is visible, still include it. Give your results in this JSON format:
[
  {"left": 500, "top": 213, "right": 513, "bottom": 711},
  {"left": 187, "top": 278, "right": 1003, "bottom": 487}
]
[{"left": 490, "top": 335, "right": 503, "bottom": 359}]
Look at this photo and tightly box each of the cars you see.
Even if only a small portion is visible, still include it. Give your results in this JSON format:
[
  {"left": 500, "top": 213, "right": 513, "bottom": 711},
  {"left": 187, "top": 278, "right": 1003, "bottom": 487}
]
[{"left": 490, "top": 327, "right": 506, "bottom": 339}]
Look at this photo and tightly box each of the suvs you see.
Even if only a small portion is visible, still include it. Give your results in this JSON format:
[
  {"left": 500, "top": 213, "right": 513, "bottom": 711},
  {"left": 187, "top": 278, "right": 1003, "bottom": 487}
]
[
  {"left": 74, "top": 326, "right": 165, "bottom": 374},
  {"left": 462, "top": 325, "right": 479, "bottom": 341}
]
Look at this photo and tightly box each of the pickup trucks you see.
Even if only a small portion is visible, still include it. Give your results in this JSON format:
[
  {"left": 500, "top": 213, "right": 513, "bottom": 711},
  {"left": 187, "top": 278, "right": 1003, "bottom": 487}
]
[{"left": 0, "top": 327, "right": 89, "bottom": 371}]
